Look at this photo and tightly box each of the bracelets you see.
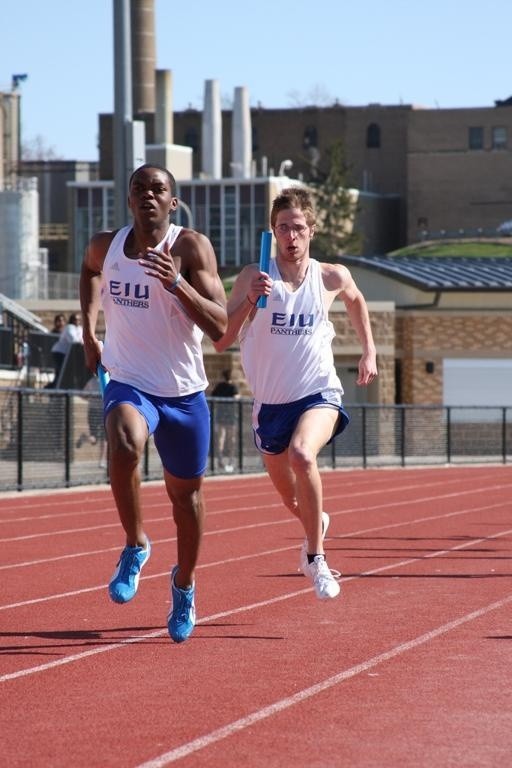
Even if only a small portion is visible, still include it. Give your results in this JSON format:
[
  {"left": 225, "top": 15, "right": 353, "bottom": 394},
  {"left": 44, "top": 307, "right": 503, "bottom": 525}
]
[
  {"left": 246, "top": 294, "right": 258, "bottom": 308},
  {"left": 166, "top": 272, "right": 183, "bottom": 295}
]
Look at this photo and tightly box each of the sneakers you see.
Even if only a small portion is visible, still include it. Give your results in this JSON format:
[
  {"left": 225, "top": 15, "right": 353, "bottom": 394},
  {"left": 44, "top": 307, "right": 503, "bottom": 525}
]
[
  {"left": 75, "top": 434, "right": 87, "bottom": 447},
  {"left": 218, "top": 461, "right": 234, "bottom": 472},
  {"left": 99, "top": 461, "right": 107, "bottom": 469},
  {"left": 300, "top": 512, "right": 329, "bottom": 575},
  {"left": 109, "top": 533, "right": 150, "bottom": 602},
  {"left": 167, "top": 566, "right": 195, "bottom": 643},
  {"left": 308, "top": 555, "right": 340, "bottom": 600}
]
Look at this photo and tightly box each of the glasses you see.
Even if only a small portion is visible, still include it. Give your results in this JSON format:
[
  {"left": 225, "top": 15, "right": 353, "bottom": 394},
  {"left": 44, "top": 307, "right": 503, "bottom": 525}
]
[{"left": 274, "top": 225, "right": 310, "bottom": 233}]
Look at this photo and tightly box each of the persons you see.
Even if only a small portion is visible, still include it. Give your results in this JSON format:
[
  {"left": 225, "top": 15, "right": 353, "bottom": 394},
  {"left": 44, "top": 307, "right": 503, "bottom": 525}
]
[
  {"left": 208, "top": 186, "right": 380, "bottom": 601},
  {"left": 78, "top": 163, "right": 232, "bottom": 649},
  {"left": 210, "top": 369, "right": 242, "bottom": 476},
  {"left": 49, "top": 313, "right": 67, "bottom": 335},
  {"left": 40, "top": 309, "right": 85, "bottom": 391}
]
